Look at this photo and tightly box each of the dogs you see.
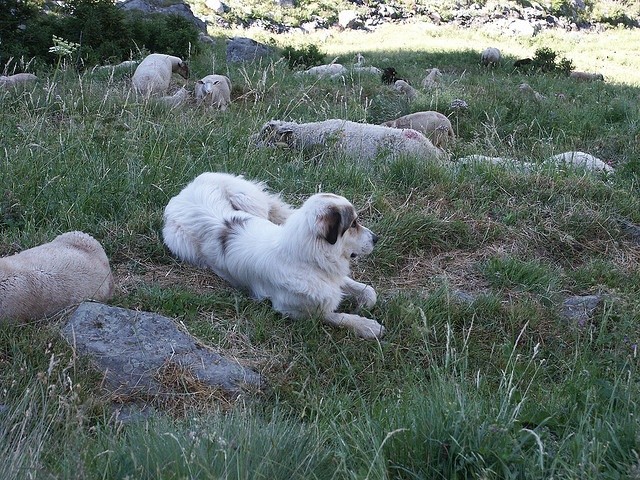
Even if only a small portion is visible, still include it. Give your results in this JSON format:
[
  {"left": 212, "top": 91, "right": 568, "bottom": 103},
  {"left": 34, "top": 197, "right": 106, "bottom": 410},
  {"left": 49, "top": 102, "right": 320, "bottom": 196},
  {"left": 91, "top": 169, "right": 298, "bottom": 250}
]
[{"left": 160, "top": 171, "right": 387, "bottom": 341}]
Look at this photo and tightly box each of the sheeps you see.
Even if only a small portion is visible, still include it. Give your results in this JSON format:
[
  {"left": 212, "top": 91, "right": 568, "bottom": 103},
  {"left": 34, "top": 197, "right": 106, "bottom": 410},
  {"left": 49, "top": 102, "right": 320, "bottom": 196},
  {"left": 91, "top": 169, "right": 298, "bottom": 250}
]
[
  {"left": 0, "top": 229, "right": 115, "bottom": 326},
  {"left": 381, "top": 67, "right": 411, "bottom": 86},
  {"left": 194, "top": 74, "right": 232, "bottom": 113},
  {"left": 458, "top": 154, "right": 537, "bottom": 176},
  {"left": 0, "top": 73, "right": 37, "bottom": 85},
  {"left": 352, "top": 52, "right": 381, "bottom": 75},
  {"left": 481, "top": 47, "right": 501, "bottom": 67},
  {"left": 131, "top": 53, "right": 191, "bottom": 100},
  {"left": 421, "top": 67, "right": 442, "bottom": 89},
  {"left": 539, "top": 151, "right": 618, "bottom": 177},
  {"left": 255, "top": 116, "right": 450, "bottom": 171},
  {"left": 297, "top": 63, "right": 347, "bottom": 80},
  {"left": 392, "top": 79, "right": 421, "bottom": 106},
  {"left": 570, "top": 71, "right": 604, "bottom": 81},
  {"left": 380, "top": 111, "right": 455, "bottom": 148}
]
